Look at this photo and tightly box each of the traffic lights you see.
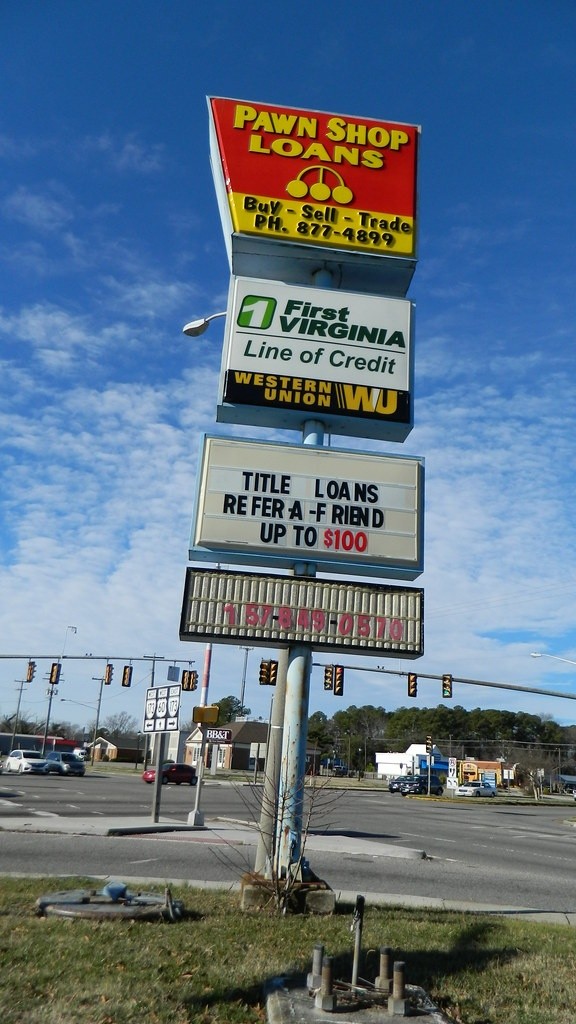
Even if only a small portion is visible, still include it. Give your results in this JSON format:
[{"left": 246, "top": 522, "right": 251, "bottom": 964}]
[
  {"left": 181, "top": 670, "right": 199, "bottom": 691},
  {"left": 425, "top": 735, "right": 433, "bottom": 753},
  {"left": 334, "top": 665, "right": 344, "bottom": 696},
  {"left": 408, "top": 672, "right": 418, "bottom": 697},
  {"left": 27, "top": 662, "right": 114, "bottom": 685},
  {"left": 324, "top": 667, "right": 334, "bottom": 690},
  {"left": 442, "top": 674, "right": 452, "bottom": 698},
  {"left": 259, "top": 660, "right": 278, "bottom": 686}
]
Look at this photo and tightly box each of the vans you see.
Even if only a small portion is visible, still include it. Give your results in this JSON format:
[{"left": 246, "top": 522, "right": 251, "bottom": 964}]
[{"left": 73, "top": 750, "right": 89, "bottom": 761}]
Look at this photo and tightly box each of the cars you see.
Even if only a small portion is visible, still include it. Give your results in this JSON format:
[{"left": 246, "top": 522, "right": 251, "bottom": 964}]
[
  {"left": 389, "top": 776, "right": 412, "bottom": 794},
  {"left": 45, "top": 752, "right": 86, "bottom": 777},
  {"left": 400, "top": 775, "right": 444, "bottom": 796},
  {"left": 455, "top": 781, "right": 497, "bottom": 798},
  {"left": 5, "top": 750, "right": 50, "bottom": 775},
  {"left": 142, "top": 763, "right": 198, "bottom": 786},
  {"left": 0, "top": 759, "right": 4, "bottom": 774}
]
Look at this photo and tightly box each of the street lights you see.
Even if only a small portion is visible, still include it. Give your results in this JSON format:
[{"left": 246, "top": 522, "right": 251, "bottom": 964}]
[
  {"left": 182, "top": 311, "right": 291, "bottom": 883},
  {"left": 61, "top": 699, "right": 99, "bottom": 765},
  {"left": 42, "top": 625, "right": 78, "bottom": 752}
]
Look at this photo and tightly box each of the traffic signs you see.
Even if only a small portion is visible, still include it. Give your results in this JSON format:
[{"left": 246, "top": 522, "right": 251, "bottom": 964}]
[{"left": 447, "top": 778, "right": 458, "bottom": 789}]
